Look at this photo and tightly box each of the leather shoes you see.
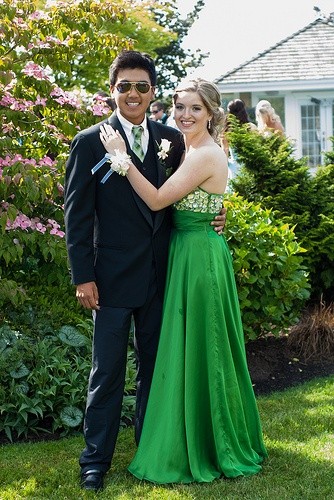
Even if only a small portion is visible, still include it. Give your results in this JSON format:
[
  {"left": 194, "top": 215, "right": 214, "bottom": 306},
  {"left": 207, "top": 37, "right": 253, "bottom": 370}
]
[{"left": 79, "top": 469, "right": 105, "bottom": 493}]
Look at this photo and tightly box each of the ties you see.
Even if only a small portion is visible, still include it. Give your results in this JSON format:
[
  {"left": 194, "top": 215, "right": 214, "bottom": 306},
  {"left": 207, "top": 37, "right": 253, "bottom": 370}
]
[{"left": 132, "top": 126, "right": 146, "bottom": 163}]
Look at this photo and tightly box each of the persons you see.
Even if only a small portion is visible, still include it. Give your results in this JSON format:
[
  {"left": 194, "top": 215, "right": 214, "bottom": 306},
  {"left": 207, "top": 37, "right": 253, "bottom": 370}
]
[
  {"left": 99, "top": 76, "right": 269, "bottom": 485},
  {"left": 148, "top": 98, "right": 285, "bottom": 192},
  {"left": 65, "top": 49, "right": 226, "bottom": 492}
]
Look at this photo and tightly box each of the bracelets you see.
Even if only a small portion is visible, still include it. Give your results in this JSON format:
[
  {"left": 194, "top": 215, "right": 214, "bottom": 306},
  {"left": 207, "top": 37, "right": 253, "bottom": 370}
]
[{"left": 91, "top": 148, "right": 131, "bottom": 183}]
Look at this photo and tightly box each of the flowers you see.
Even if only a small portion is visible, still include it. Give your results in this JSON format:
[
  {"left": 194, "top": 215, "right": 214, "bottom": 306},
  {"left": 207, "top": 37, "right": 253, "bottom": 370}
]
[{"left": 155, "top": 138, "right": 174, "bottom": 164}]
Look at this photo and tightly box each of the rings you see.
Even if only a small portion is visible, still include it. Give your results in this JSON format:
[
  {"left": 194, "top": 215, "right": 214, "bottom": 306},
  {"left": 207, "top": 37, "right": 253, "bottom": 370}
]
[{"left": 106, "top": 132, "right": 113, "bottom": 138}]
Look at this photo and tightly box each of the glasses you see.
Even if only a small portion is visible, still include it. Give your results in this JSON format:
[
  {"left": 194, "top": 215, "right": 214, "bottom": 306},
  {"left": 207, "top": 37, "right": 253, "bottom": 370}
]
[
  {"left": 150, "top": 109, "right": 162, "bottom": 115},
  {"left": 114, "top": 81, "right": 153, "bottom": 94}
]
